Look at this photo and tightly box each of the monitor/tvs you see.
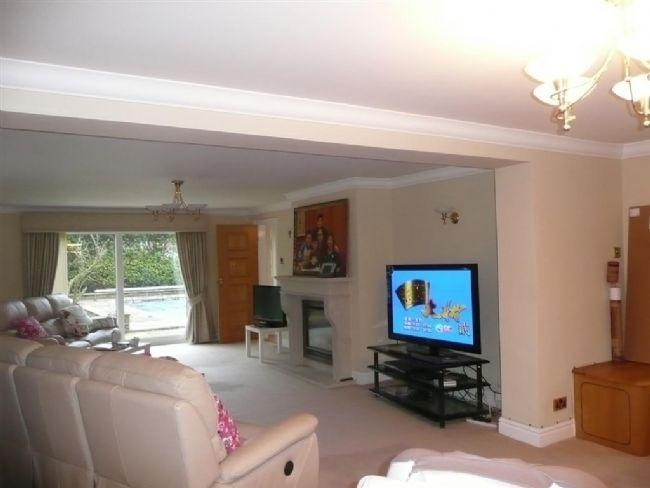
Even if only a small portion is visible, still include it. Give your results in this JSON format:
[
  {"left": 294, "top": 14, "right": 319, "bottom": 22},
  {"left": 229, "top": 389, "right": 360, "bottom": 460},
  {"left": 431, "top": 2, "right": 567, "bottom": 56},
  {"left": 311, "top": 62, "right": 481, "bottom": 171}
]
[
  {"left": 251, "top": 284, "right": 287, "bottom": 328},
  {"left": 386, "top": 262, "right": 483, "bottom": 359}
]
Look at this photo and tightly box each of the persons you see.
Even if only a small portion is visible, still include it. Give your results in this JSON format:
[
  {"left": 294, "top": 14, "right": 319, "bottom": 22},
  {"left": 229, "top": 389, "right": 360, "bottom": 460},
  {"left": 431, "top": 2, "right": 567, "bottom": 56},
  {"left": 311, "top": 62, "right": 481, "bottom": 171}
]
[{"left": 297, "top": 213, "right": 345, "bottom": 274}]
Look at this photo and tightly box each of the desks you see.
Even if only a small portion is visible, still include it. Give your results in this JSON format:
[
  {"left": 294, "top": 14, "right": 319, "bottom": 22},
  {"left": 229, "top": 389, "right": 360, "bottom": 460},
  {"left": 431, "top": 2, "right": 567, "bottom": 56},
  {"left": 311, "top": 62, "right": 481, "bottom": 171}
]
[{"left": 243, "top": 324, "right": 290, "bottom": 364}]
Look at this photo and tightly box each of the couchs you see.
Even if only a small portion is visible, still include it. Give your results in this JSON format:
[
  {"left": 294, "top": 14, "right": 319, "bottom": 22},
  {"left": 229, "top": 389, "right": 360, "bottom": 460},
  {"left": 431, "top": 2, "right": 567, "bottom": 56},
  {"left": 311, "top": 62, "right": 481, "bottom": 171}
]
[{"left": 0, "top": 293, "right": 320, "bottom": 488}]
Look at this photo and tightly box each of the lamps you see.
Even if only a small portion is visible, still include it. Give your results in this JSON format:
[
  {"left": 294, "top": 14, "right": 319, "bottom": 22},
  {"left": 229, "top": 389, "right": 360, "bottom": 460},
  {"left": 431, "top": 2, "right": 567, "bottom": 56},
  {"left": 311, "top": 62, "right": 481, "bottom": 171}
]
[{"left": 146, "top": 179, "right": 207, "bottom": 223}]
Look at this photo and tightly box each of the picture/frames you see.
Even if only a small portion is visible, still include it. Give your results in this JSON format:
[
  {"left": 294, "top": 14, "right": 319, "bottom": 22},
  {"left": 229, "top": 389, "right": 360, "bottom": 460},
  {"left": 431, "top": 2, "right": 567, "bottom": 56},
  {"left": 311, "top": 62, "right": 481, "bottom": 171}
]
[{"left": 293, "top": 197, "right": 349, "bottom": 276}]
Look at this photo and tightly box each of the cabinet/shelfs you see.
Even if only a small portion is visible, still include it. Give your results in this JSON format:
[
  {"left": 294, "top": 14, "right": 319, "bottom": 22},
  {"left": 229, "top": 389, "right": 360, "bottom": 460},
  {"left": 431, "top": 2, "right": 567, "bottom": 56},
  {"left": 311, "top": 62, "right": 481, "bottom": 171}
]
[
  {"left": 366, "top": 342, "right": 491, "bottom": 428},
  {"left": 573, "top": 361, "right": 650, "bottom": 457}
]
